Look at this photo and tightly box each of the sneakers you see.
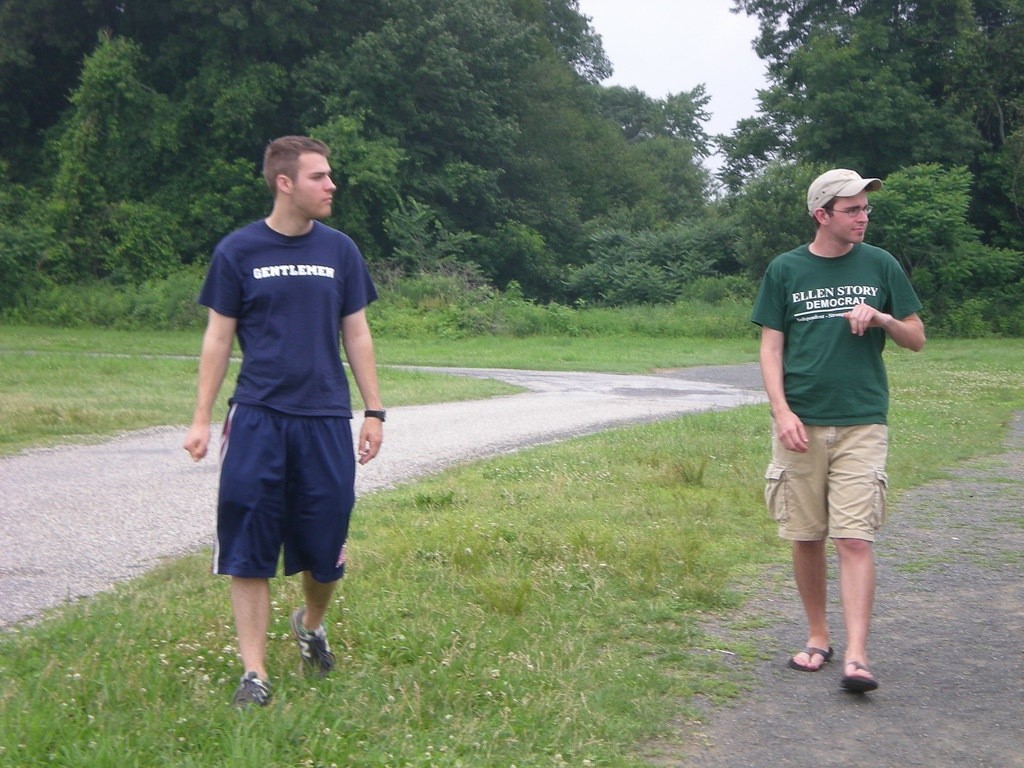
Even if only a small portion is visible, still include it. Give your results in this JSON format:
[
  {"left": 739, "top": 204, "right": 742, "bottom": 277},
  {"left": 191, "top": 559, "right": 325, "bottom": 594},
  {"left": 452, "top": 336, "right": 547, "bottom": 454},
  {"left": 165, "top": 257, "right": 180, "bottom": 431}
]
[
  {"left": 288, "top": 609, "right": 337, "bottom": 686},
  {"left": 229, "top": 670, "right": 275, "bottom": 714}
]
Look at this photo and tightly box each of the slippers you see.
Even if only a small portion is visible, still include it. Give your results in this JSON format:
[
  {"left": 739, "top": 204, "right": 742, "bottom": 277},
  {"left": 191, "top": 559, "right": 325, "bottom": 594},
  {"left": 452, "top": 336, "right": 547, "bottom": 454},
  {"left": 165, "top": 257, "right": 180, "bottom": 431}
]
[
  {"left": 787, "top": 645, "right": 834, "bottom": 672},
  {"left": 837, "top": 662, "right": 879, "bottom": 693}
]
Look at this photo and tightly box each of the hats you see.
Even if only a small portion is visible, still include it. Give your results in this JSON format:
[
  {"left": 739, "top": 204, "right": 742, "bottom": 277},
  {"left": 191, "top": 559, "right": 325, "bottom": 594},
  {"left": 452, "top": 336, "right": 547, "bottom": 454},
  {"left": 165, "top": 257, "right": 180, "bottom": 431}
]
[{"left": 806, "top": 168, "right": 883, "bottom": 218}]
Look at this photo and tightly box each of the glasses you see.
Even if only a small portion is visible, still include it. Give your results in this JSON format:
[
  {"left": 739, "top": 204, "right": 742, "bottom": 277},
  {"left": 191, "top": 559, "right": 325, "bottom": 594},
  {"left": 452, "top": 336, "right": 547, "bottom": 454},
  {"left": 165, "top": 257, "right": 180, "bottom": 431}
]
[{"left": 828, "top": 205, "right": 874, "bottom": 217}]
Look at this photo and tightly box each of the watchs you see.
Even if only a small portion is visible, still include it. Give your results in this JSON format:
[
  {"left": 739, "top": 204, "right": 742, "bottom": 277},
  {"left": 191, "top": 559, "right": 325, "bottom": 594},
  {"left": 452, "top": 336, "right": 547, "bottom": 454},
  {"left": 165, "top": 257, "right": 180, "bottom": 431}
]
[{"left": 365, "top": 410, "right": 385, "bottom": 422}]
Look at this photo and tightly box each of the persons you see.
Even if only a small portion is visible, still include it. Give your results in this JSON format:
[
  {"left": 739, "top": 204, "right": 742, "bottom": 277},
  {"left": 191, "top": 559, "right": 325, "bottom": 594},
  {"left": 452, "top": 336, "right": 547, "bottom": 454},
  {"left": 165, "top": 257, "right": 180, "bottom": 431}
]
[
  {"left": 751, "top": 169, "right": 925, "bottom": 691},
  {"left": 184, "top": 136, "right": 386, "bottom": 706}
]
